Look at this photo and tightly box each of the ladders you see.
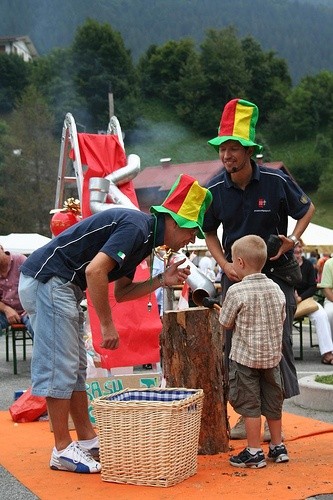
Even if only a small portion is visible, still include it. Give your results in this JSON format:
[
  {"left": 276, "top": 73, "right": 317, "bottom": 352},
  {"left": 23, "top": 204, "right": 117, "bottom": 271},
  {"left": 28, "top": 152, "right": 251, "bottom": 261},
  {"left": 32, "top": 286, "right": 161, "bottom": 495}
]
[{"left": 53, "top": 112, "right": 127, "bottom": 237}]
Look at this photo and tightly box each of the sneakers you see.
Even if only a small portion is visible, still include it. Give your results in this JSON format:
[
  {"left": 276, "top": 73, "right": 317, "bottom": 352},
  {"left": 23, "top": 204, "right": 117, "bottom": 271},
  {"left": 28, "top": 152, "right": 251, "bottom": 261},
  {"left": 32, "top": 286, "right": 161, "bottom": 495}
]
[
  {"left": 263, "top": 420, "right": 285, "bottom": 443},
  {"left": 48, "top": 439, "right": 103, "bottom": 472},
  {"left": 229, "top": 417, "right": 248, "bottom": 439},
  {"left": 76, "top": 437, "right": 101, "bottom": 462},
  {"left": 268, "top": 442, "right": 290, "bottom": 463},
  {"left": 229, "top": 447, "right": 268, "bottom": 471}
]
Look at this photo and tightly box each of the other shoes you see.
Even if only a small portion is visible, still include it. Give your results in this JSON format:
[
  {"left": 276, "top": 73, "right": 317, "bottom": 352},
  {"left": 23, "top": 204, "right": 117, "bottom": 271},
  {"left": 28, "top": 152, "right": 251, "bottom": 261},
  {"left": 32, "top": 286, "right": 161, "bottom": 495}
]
[{"left": 322, "top": 355, "right": 333, "bottom": 365}]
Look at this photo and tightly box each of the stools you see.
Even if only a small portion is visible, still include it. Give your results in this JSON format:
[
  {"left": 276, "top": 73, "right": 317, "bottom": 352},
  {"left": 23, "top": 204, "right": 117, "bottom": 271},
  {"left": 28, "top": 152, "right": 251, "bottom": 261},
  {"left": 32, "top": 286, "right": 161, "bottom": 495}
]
[
  {"left": 5, "top": 323, "right": 32, "bottom": 375},
  {"left": 293, "top": 316, "right": 319, "bottom": 361}
]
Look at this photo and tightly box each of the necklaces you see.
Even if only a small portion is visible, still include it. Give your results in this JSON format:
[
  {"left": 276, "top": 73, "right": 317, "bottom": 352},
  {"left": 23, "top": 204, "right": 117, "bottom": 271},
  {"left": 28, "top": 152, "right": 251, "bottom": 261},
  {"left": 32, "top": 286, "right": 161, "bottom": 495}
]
[{"left": 147, "top": 212, "right": 157, "bottom": 311}]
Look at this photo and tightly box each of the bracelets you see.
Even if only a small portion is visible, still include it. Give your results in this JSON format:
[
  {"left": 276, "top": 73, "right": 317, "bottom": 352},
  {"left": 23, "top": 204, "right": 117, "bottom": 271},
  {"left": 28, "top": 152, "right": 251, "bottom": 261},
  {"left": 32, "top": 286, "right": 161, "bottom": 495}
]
[{"left": 157, "top": 273, "right": 165, "bottom": 286}]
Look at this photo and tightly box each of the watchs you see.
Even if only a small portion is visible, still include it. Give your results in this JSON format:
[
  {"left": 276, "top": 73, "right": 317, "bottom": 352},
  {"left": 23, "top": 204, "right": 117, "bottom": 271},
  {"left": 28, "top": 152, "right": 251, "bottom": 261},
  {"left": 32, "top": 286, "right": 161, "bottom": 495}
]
[{"left": 287, "top": 234, "right": 300, "bottom": 247}]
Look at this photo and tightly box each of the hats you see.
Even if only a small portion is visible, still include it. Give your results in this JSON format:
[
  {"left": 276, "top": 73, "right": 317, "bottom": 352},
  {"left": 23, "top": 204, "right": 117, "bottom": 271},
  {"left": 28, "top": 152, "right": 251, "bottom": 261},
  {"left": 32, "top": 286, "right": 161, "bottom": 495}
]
[
  {"left": 149, "top": 173, "right": 213, "bottom": 239},
  {"left": 208, "top": 98, "right": 264, "bottom": 153}
]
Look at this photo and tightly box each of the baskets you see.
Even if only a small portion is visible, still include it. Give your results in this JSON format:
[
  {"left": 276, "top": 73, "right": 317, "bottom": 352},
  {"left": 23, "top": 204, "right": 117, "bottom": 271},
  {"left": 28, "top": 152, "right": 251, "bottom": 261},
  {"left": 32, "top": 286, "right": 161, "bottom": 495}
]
[{"left": 94, "top": 385, "right": 205, "bottom": 488}]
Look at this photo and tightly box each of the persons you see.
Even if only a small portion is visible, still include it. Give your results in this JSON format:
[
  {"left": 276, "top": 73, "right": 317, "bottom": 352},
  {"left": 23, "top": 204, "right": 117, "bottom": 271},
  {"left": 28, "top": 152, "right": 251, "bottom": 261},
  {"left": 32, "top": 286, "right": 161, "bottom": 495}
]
[
  {"left": 18, "top": 172, "right": 213, "bottom": 474},
  {"left": 202, "top": 98, "right": 315, "bottom": 442},
  {"left": 198, "top": 238, "right": 333, "bottom": 365},
  {"left": 212, "top": 234, "right": 290, "bottom": 468},
  {"left": 0, "top": 244, "right": 34, "bottom": 347}
]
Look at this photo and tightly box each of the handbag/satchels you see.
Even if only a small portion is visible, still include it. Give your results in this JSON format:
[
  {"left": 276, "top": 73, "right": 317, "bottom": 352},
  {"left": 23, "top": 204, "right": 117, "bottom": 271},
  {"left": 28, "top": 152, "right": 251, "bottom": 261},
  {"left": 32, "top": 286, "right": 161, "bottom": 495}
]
[
  {"left": 273, "top": 259, "right": 302, "bottom": 286},
  {"left": 294, "top": 297, "right": 318, "bottom": 319}
]
